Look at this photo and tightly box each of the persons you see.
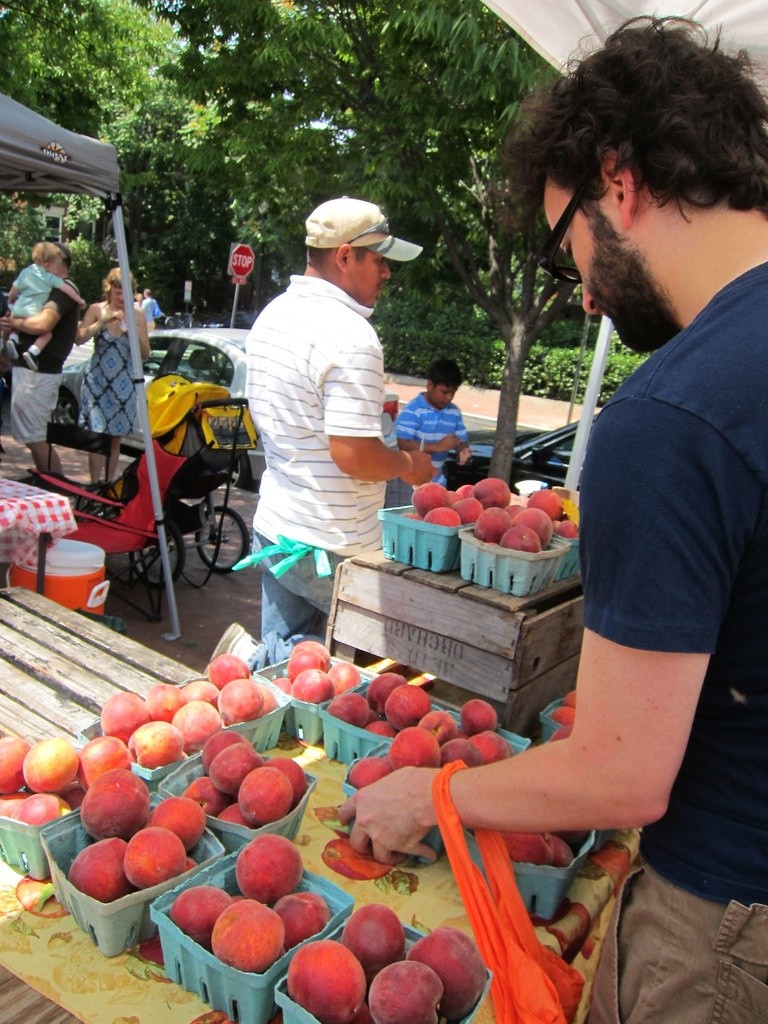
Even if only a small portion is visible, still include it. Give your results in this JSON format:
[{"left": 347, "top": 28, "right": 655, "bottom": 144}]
[
  {"left": 201, "top": 196, "right": 441, "bottom": 674},
  {"left": 0, "top": 293, "right": 11, "bottom": 454},
  {"left": 134, "top": 293, "right": 143, "bottom": 308},
  {"left": 0, "top": 243, "right": 81, "bottom": 485},
  {"left": 141, "top": 288, "right": 166, "bottom": 331},
  {"left": 396, "top": 358, "right": 472, "bottom": 491},
  {"left": 339, "top": 5, "right": 768, "bottom": 1024},
  {"left": 6, "top": 242, "right": 86, "bottom": 371},
  {"left": 75, "top": 268, "right": 150, "bottom": 483}
]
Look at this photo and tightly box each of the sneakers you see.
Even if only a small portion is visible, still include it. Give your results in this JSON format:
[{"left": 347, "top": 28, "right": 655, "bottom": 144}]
[
  {"left": 205, "top": 622, "right": 261, "bottom": 677},
  {"left": 24, "top": 351, "right": 39, "bottom": 371},
  {"left": 7, "top": 338, "right": 21, "bottom": 360}
]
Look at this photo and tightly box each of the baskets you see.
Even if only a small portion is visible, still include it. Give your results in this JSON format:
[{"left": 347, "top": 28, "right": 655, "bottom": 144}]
[
  {"left": 0, "top": 651, "right": 618, "bottom": 1024},
  {"left": 379, "top": 505, "right": 583, "bottom": 597}
]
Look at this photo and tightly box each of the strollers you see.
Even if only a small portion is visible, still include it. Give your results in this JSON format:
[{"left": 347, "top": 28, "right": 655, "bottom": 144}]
[{"left": 20, "top": 371, "right": 251, "bottom": 582}]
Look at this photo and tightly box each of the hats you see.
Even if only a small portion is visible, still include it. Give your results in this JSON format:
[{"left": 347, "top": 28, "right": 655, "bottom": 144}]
[{"left": 305, "top": 196, "right": 423, "bottom": 262}]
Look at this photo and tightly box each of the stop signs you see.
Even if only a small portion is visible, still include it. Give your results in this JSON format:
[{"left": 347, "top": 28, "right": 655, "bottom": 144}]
[{"left": 230, "top": 244, "right": 255, "bottom": 278}]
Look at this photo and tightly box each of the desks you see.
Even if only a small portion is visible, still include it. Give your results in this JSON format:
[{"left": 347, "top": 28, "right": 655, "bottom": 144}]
[
  {"left": 1, "top": 585, "right": 645, "bottom": 1024},
  {"left": 0, "top": 476, "right": 78, "bottom": 598},
  {"left": 325, "top": 548, "right": 585, "bottom": 747}
]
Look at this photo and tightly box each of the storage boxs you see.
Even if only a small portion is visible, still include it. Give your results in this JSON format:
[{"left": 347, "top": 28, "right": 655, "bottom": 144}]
[{"left": 1, "top": 500, "right": 622, "bottom": 1024}]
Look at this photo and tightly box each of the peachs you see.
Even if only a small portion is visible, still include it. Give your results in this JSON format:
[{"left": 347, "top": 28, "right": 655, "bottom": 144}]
[
  {"left": 400, "top": 478, "right": 578, "bottom": 554},
  {"left": 0, "top": 637, "right": 580, "bottom": 1024}
]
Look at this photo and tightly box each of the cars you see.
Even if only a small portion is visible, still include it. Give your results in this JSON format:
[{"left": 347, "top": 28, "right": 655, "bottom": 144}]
[
  {"left": 441, "top": 414, "right": 599, "bottom": 496},
  {"left": 58, "top": 327, "right": 268, "bottom": 489}
]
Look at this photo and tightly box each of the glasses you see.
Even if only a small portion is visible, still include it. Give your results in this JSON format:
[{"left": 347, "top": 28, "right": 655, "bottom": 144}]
[
  {"left": 336, "top": 217, "right": 390, "bottom": 255},
  {"left": 536, "top": 163, "right": 598, "bottom": 284}
]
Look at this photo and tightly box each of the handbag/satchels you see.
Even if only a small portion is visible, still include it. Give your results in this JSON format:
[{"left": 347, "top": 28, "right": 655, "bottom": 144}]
[
  {"left": 433, "top": 759, "right": 586, "bottom": 1024},
  {"left": 152, "top": 299, "right": 162, "bottom": 318}
]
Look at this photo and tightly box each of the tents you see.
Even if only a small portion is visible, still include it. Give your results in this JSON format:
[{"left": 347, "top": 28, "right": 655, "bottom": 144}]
[
  {"left": 483, "top": 0, "right": 768, "bottom": 502},
  {"left": 0, "top": 92, "right": 181, "bottom": 642}
]
